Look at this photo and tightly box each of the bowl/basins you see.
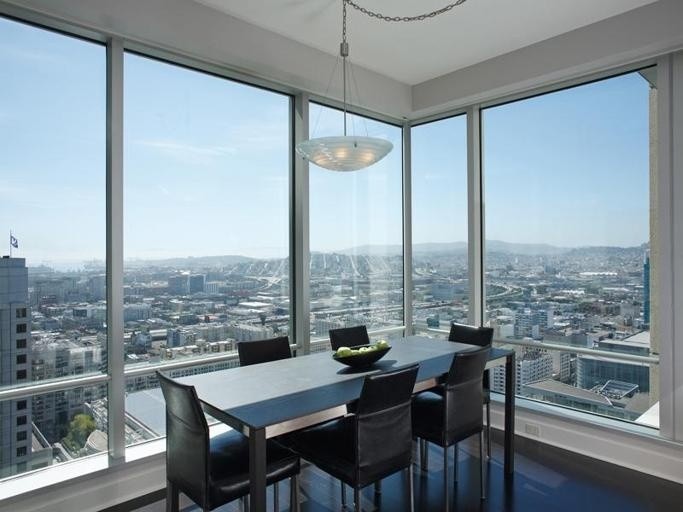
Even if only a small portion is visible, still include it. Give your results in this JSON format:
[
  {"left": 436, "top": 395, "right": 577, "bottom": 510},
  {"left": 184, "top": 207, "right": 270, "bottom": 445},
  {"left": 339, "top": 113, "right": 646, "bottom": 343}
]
[{"left": 332, "top": 343, "right": 391, "bottom": 370}]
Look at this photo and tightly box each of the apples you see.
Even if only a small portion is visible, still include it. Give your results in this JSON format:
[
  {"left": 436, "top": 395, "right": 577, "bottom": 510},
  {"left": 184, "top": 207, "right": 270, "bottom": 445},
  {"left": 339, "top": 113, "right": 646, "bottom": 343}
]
[{"left": 337, "top": 339, "right": 389, "bottom": 357}]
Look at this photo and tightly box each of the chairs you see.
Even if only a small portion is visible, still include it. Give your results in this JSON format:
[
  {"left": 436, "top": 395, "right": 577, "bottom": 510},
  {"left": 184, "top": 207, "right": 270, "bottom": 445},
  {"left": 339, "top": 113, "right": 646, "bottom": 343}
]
[
  {"left": 328, "top": 324, "right": 381, "bottom": 414},
  {"left": 432, "top": 322, "right": 495, "bottom": 457},
  {"left": 280, "top": 362, "right": 420, "bottom": 511},
  {"left": 411, "top": 343, "right": 491, "bottom": 510},
  {"left": 235, "top": 335, "right": 291, "bottom": 367},
  {"left": 154, "top": 370, "right": 301, "bottom": 512}
]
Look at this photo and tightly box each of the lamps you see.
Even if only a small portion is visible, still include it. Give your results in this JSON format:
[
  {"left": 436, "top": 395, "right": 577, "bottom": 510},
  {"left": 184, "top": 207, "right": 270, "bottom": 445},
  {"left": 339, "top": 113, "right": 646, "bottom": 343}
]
[{"left": 292, "top": 0, "right": 466, "bottom": 173}]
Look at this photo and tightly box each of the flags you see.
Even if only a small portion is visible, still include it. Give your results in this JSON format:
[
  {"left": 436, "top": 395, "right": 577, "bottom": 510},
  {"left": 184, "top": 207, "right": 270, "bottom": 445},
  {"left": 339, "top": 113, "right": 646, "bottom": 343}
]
[{"left": 8, "top": 230, "right": 18, "bottom": 257}]
